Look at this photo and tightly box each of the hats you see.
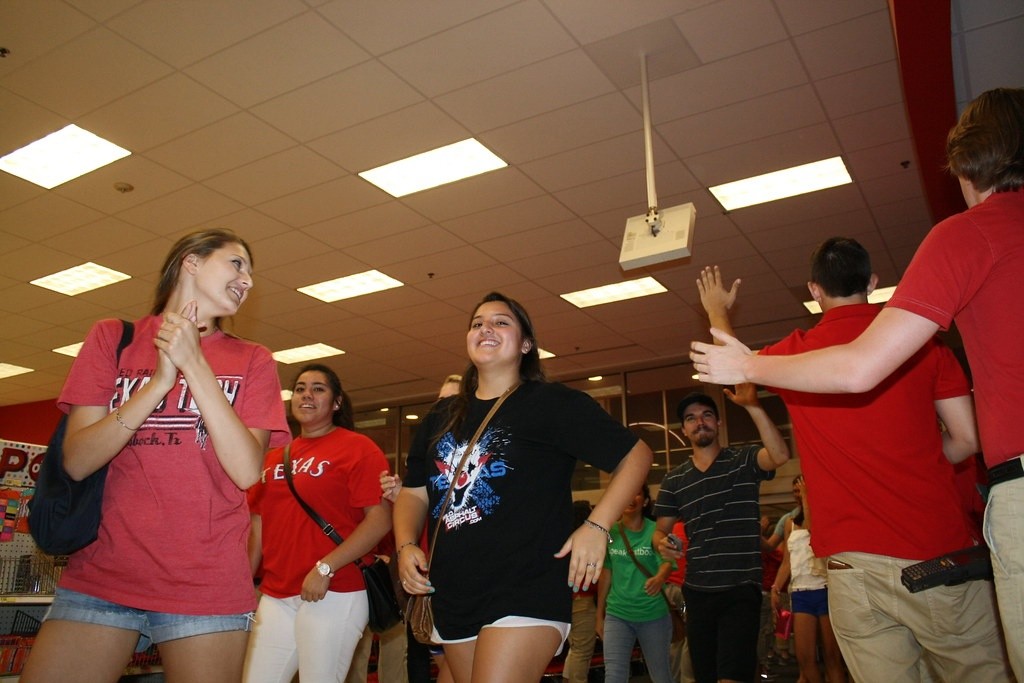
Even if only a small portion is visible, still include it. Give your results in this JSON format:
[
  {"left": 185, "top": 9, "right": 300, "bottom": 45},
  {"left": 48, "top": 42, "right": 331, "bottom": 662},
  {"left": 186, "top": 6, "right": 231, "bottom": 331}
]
[{"left": 675, "top": 392, "right": 719, "bottom": 427}]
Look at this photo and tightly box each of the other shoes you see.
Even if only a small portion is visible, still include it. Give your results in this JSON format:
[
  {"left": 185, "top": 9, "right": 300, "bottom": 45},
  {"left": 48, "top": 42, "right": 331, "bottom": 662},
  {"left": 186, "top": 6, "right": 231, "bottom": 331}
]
[
  {"left": 761, "top": 672, "right": 781, "bottom": 682},
  {"left": 766, "top": 651, "right": 777, "bottom": 660},
  {"left": 779, "top": 654, "right": 791, "bottom": 662}
]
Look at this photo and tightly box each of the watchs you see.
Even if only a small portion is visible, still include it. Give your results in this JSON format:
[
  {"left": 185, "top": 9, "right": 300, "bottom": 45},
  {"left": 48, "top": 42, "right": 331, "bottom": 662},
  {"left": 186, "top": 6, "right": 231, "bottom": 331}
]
[{"left": 316, "top": 560, "right": 334, "bottom": 578}]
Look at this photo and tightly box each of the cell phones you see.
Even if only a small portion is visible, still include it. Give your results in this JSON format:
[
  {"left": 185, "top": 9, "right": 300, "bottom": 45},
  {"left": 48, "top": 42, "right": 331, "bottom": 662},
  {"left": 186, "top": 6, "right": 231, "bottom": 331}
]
[{"left": 667, "top": 533, "right": 683, "bottom": 552}]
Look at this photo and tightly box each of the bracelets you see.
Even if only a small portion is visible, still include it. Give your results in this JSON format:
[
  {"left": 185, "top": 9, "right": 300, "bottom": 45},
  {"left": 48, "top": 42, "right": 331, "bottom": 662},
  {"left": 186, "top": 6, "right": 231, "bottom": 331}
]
[
  {"left": 396, "top": 542, "right": 418, "bottom": 563},
  {"left": 116, "top": 408, "right": 140, "bottom": 431},
  {"left": 584, "top": 519, "right": 613, "bottom": 544}
]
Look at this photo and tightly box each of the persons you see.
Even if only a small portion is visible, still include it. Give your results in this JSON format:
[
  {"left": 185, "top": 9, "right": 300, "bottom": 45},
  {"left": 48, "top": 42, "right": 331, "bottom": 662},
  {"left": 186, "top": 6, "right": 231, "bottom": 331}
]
[
  {"left": 689, "top": 88, "right": 1024, "bottom": 683},
  {"left": 652, "top": 383, "right": 790, "bottom": 683},
  {"left": 561, "top": 482, "right": 689, "bottom": 683},
  {"left": 760, "top": 474, "right": 846, "bottom": 683},
  {"left": 696, "top": 237, "right": 982, "bottom": 683},
  {"left": 18, "top": 228, "right": 292, "bottom": 683},
  {"left": 380, "top": 291, "right": 652, "bottom": 683},
  {"left": 242, "top": 363, "right": 393, "bottom": 683}
]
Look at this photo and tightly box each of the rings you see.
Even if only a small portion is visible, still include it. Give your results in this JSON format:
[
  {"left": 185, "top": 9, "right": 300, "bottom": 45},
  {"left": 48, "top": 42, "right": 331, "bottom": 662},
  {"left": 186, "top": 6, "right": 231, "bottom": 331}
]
[
  {"left": 403, "top": 578, "right": 406, "bottom": 585},
  {"left": 587, "top": 563, "right": 596, "bottom": 568}
]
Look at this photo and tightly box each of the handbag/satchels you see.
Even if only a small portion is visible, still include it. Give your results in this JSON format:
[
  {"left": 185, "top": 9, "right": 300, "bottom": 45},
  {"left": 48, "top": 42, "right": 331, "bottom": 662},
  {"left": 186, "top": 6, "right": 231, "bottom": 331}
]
[
  {"left": 671, "top": 609, "right": 687, "bottom": 643},
  {"left": 773, "top": 607, "right": 793, "bottom": 642},
  {"left": 26, "top": 319, "right": 135, "bottom": 555},
  {"left": 359, "top": 558, "right": 408, "bottom": 634},
  {"left": 403, "top": 574, "right": 434, "bottom": 644}
]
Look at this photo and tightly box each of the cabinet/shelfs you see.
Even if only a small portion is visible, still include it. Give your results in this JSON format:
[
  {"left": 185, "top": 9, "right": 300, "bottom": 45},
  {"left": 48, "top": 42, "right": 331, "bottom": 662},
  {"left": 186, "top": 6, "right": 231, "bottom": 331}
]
[{"left": 0, "top": 438, "right": 164, "bottom": 683}]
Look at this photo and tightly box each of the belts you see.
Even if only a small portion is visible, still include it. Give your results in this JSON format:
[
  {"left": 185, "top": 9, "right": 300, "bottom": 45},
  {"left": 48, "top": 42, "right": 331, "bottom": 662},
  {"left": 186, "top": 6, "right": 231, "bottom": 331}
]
[{"left": 986, "top": 459, "right": 1023, "bottom": 491}]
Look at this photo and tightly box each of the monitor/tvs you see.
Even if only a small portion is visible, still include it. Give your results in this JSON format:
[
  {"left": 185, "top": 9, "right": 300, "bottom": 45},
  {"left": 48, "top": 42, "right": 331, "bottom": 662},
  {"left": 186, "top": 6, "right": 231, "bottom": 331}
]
[{"left": 619, "top": 203, "right": 697, "bottom": 272}]
